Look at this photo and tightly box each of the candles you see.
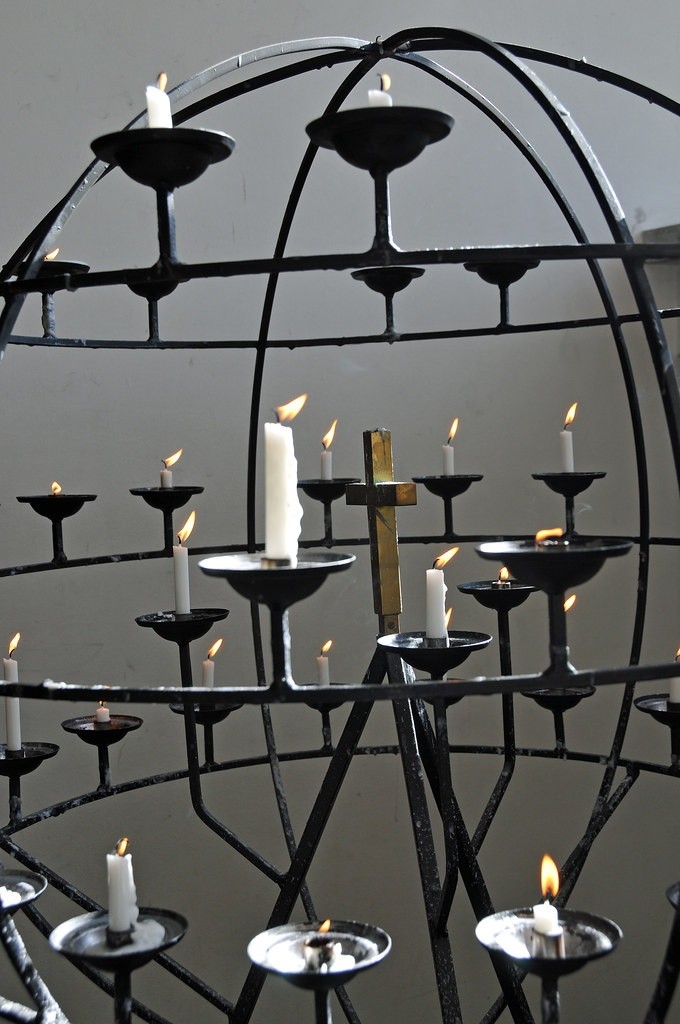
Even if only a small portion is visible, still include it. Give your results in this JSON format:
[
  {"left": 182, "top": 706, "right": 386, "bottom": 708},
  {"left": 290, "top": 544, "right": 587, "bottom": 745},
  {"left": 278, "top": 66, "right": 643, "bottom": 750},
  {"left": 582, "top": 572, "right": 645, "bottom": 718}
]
[
  {"left": 320, "top": 441, "right": 333, "bottom": 481},
  {"left": 202, "top": 654, "right": 214, "bottom": 688},
  {"left": 106, "top": 839, "right": 139, "bottom": 931},
  {"left": 172, "top": 535, "right": 190, "bottom": 614},
  {"left": 425, "top": 558, "right": 446, "bottom": 638},
  {"left": 533, "top": 888, "right": 559, "bottom": 933},
  {"left": 561, "top": 424, "right": 576, "bottom": 472},
  {"left": 4, "top": 648, "right": 20, "bottom": 751},
  {"left": 317, "top": 650, "right": 330, "bottom": 686},
  {"left": 146, "top": 84, "right": 172, "bottom": 129},
  {"left": 159, "top": 460, "right": 172, "bottom": 488},
  {"left": 442, "top": 436, "right": 455, "bottom": 477},
  {"left": 98, "top": 702, "right": 108, "bottom": 720},
  {"left": 264, "top": 409, "right": 303, "bottom": 559},
  {"left": 669, "top": 654, "right": 680, "bottom": 703},
  {"left": 368, "top": 73, "right": 393, "bottom": 107}
]
